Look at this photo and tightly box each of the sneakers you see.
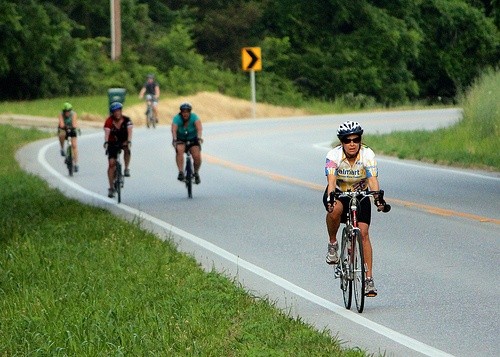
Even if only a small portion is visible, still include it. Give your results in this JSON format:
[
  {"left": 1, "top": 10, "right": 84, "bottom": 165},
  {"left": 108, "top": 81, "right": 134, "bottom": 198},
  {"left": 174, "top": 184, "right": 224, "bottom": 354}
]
[
  {"left": 325, "top": 241, "right": 340, "bottom": 265},
  {"left": 365, "top": 277, "right": 377, "bottom": 297}
]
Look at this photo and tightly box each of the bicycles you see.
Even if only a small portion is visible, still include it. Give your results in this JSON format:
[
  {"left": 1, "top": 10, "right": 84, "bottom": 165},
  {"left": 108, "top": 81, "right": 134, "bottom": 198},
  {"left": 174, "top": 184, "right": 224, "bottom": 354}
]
[
  {"left": 328, "top": 188, "right": 385, "bottom": 313},
  {"left": 103, "top": 141, "right": 130, "bottom": 203},
  {"left": 58, "top": 126, "right": 81, "bottom": 175},
  {"left": 142, "top": 95, "right": 158, "bottom": 129},
  {"left": 173, "top": 138, "right": 199, "bottom": 198}
]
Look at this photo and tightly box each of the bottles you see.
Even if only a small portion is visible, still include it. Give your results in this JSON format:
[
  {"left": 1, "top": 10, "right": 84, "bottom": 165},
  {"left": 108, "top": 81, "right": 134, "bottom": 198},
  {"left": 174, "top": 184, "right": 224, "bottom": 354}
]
[{"left": 348, "top": 233, "right": 352, "bottom": 256}]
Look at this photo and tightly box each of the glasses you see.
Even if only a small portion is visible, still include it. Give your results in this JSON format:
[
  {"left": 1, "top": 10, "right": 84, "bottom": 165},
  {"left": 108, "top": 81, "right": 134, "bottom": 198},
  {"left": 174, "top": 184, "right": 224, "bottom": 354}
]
[{"left": 343, "top": 137, "right": 361, "bottom": 144}]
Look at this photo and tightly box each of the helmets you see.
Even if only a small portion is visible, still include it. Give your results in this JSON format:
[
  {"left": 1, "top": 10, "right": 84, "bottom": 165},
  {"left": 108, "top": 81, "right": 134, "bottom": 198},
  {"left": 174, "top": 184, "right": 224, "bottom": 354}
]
[
  {"left": 180, "top": 103, "right": 192, "bottom": 110},
  {"left": 336, "top": 121, "right": 363, "bottom": 136},
  {"left": 62, "top": 103, "right": 72, "bottom": 111},
  {"left": 110, "top": 102, "right": 122, "bottom": 112}
]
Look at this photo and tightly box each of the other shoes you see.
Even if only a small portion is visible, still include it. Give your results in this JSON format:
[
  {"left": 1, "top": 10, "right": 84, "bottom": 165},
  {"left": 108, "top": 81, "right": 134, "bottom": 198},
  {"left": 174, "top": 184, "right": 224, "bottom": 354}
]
[
  {"left": 195, "top": 176, "right": 200, "bottom": 184},
  {"left": 108, "top": 188, "right": 114, "bottom": 198},
  {"left": 74, "top": 166, "right": 79, "bottom": 172},
  {"left": 178, "top": 171, "right": 184, "bottom": 180},
  {"left": 61, "top": 151, "right": 65, "bottom": 156},
  {"left": 124, "top": 169, "right": 130, "bottom": 177}
]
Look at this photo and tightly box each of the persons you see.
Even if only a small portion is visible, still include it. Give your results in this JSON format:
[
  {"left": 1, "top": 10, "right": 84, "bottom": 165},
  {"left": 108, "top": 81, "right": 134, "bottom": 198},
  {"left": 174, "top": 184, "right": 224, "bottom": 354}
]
[
  {"left": 171, "top": 102, "right": 203, "bottom": 184},
  {"left": 58, "top": 103, "right": 79, "bottom": 173},
  {"left": 322, "top": 121, "right": 386, "bottom": 296},
  {"left": 102, "top": 102, "right": 134, "bottom": 198},
  {"left": 139, "top": 73, "right": 160, "bottom": 123}
]
